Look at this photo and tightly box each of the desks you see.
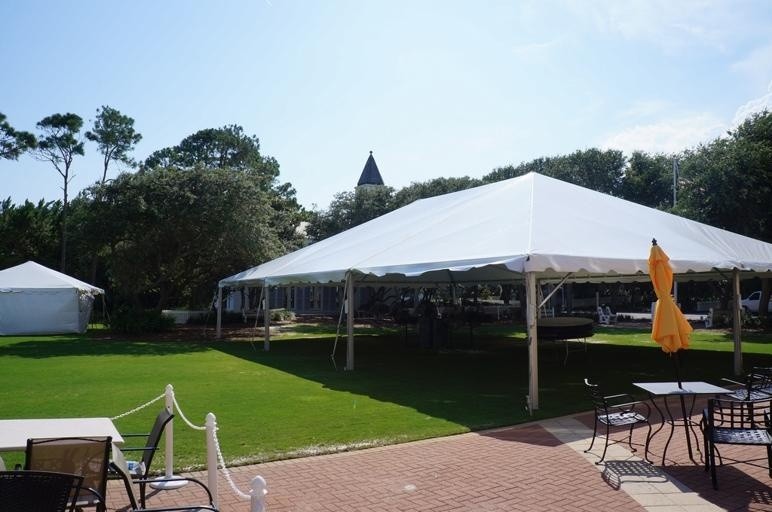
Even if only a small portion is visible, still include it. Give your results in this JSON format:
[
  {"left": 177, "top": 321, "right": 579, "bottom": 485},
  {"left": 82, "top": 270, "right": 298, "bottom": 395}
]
[{"left": 537, "top": 316, "right": 596, "bottom": 361}]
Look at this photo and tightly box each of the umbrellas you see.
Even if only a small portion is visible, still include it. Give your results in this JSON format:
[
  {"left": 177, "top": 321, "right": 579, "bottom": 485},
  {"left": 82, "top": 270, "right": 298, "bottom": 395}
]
[{"left": 649, "top": 239, "right": 693, "bottom": 461}]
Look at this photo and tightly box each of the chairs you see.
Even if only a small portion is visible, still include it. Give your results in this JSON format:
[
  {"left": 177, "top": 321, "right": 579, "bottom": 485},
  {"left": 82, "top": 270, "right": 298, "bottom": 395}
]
[
  {"left": 110, "top": 442, "right": 221, "bottom": 512},
  {"left": 582, "top": 377, "right": 654, "bottom": 466},
  {"left": 596, "top": 305, "right": 619, "bottom": 326},
  {"left": 1, "top": 469, "right": 87, "bottom": 512},
  {"left": 13, "top": 435, "right": 113, "bottom": 511},
  {"left": 100, "top": 406, "right": 175, "bottom": 511},
  {"left": 717, "top": 366, "right": 772, "bottom": 430},
  {"left": 697, "top": 397, "right": 772, "bottom": 491}
]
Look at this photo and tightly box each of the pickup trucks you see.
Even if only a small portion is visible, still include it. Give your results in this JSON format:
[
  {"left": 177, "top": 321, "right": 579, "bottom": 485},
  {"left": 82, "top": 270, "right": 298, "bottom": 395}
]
[{"left": 741, "top": 291, "right": 772, "bottom": 314}]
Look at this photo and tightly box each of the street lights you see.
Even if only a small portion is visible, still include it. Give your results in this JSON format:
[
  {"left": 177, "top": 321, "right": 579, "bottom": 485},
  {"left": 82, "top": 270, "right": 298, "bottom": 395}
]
[{"left": 664, "top": 158, "right": 688, "bottom": 305}]
